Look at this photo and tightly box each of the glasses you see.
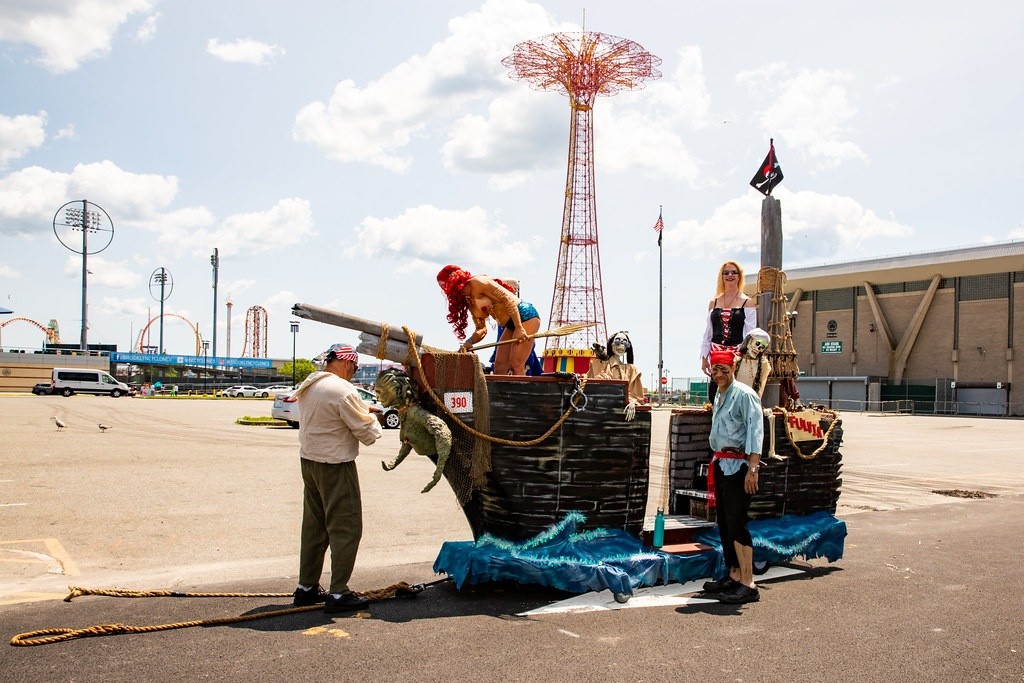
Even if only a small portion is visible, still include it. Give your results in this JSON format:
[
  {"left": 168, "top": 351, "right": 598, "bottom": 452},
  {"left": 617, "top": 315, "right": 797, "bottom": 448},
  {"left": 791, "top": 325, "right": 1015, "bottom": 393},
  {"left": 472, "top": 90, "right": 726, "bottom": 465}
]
[
  {"left": 724, "top": 270, "right": 738, "bottom": 275},
  {"left": 352, "top": 361, "right": 358, "bottom": 374}
]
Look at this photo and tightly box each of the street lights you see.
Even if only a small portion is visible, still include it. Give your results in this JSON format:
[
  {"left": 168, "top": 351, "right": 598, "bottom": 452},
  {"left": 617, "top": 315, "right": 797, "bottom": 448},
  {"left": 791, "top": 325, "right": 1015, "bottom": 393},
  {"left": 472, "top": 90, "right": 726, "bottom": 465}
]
[
  {"left": 664, "top": 368, "right": 670, "bottom": 398},
  {"left": 786, "top": 311, "right": 798, "bottom": 345},
  {"left": 290, "top": 319, "right": 300, "bottom": 386}
]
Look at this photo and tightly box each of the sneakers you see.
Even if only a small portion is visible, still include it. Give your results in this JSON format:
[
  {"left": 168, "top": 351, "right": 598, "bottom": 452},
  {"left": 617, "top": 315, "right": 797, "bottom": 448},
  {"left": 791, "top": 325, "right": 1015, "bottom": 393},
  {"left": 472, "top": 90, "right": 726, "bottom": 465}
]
[
  {"left": 294, "top": 582, "right": 327, "bottom": 607},
  {"left": 324, "top": 591, "right": 369, "bottom": 613}
]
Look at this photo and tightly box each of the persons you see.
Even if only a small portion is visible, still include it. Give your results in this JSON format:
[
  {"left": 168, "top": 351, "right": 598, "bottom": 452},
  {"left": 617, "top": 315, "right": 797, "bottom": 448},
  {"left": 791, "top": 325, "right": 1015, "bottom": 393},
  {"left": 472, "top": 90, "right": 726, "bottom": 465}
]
[
  {"left": 724, "top": 291, "right": 738, "bottom": 307},
  {"left": 174, "top": 384, "right": 178, "bottom": 396},
  {"left": 703, "top": 352, "right": 764, "bottom": 603},
  {"left": 288, "top": 343, "right": 383, "bottom": 612},
  {"left": 161, "top": 382, "right": 166, "bottom": 396},
  {"left": 141, "top": 383, "right": 155, "bottom": 396},
  {"left": 437, "top": 265, "right": 540, "bottom": 375},
  {"left": 701, "top": 262, "right": 757, "bottom": 410}
]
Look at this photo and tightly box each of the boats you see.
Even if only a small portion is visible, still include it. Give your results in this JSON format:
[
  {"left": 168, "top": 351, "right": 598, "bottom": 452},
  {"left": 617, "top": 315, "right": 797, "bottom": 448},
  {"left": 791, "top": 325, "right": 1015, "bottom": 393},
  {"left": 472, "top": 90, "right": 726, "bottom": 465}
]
[{"left": 292, "top": 300, "right": 848, "bottom": 599}]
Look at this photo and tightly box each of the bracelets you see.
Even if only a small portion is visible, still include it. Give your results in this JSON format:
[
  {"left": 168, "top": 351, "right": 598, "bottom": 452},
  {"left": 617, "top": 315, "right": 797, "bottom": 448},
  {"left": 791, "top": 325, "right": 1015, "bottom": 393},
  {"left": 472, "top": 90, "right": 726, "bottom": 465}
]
[{"left": 468, "top": 338, "right": 473, "bottom": 344}]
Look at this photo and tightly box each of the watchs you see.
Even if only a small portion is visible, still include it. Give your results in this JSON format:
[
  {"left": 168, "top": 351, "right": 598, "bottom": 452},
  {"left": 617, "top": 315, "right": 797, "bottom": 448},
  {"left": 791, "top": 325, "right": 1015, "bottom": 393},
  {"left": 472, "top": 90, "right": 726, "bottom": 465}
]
[{"left": 749, "top": 466, "right": 758, "bottom": 473}]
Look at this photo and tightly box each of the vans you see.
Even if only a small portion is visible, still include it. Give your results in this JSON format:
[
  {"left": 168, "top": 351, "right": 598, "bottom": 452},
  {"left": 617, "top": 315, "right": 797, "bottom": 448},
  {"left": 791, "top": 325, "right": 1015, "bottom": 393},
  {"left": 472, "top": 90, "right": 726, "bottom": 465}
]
[{"left": 49, "top": 368, "right": 131, "bottom": 398}]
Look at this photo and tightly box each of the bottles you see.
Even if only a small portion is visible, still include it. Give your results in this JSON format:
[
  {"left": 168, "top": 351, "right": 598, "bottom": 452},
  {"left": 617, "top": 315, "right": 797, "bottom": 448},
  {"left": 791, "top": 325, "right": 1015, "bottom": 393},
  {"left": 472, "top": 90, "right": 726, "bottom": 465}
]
[{"left": 653, "top": 506, "right": 664, "bottom": 548}]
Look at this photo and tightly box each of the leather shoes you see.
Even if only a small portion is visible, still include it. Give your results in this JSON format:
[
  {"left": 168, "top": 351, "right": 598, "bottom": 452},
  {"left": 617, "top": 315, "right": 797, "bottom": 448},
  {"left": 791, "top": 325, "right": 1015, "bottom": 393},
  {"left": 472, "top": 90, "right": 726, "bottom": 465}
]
[
  {"left": 716, "top": 580, "right": 760, "bottom": 603},
  {"left": 703, "top": 574, "right": 740, "bottom": 593}
]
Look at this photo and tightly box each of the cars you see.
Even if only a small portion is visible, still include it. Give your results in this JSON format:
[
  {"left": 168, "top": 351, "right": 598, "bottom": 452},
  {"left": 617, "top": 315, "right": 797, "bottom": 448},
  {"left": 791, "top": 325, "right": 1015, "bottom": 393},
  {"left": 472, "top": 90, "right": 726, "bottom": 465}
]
[
  {"left": 33, "top": 382, "right": 69, "bottom": 396},
  {"left": 214, "top": 384, "right": 294, "bottom": 398},
  {"left": 269, "top": 386, "right": 401, "bottom": 430}
]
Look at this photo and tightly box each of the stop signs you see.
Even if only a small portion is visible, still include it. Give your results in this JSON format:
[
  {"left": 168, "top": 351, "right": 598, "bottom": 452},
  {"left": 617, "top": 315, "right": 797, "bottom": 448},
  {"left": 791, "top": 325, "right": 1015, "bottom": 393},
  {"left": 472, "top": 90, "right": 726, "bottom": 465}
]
[{"left": 661, "top": 377, "right": 667, "bottom": 384}]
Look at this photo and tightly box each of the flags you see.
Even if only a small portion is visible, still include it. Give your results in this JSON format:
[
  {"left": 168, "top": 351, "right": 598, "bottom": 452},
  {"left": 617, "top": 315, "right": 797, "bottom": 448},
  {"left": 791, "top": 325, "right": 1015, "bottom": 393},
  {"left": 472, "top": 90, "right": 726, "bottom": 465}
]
[
  {"left": 654, "top": 213, "right": 664, "bottom": 233},
  {"left": 749, "top": 145, "right": 784, "bottom": 196}
]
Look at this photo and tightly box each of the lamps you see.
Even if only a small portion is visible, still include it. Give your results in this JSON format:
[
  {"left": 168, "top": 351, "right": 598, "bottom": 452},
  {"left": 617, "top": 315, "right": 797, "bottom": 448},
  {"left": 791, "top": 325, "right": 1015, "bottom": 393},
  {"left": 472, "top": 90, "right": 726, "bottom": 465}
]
[
  {"left": 977, "top": 347, "right": 986, "bottom": 360},
  {"left": 870, "top": 323, "right": 878, "bottom": 333}
]
